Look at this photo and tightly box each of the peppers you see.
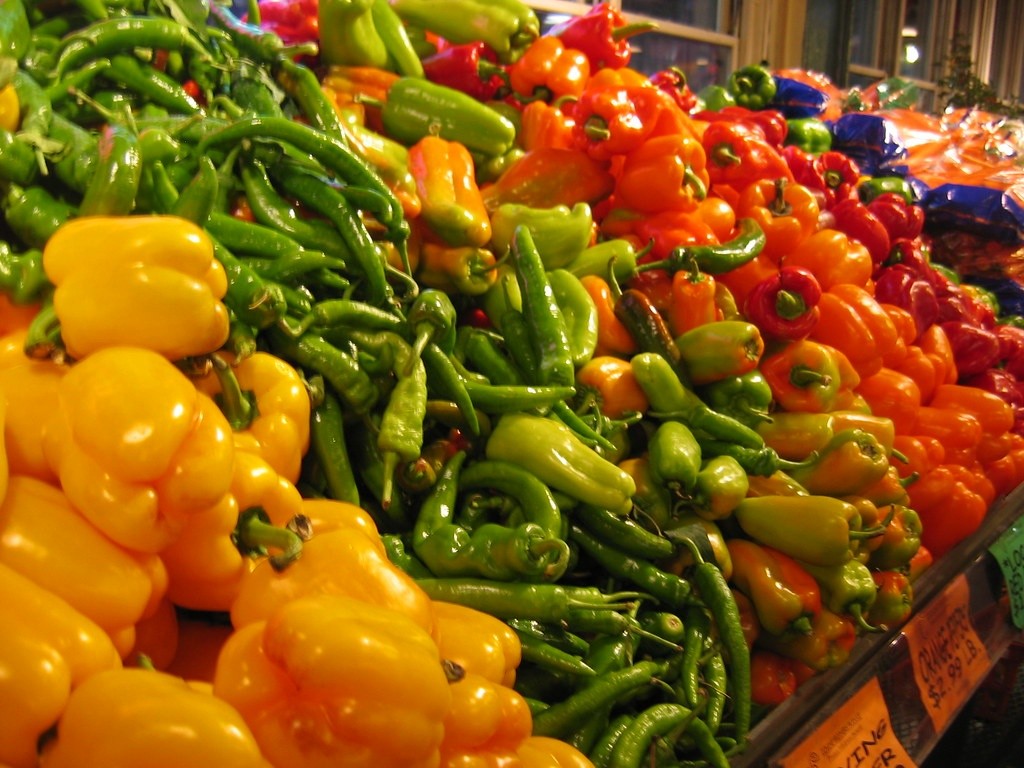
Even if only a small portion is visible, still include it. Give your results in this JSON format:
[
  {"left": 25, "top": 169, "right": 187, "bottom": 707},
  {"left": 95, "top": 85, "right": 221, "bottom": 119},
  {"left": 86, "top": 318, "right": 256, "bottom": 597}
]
[{"left": 0, "top": 0, "right": 1024, "bottom": 768}]
